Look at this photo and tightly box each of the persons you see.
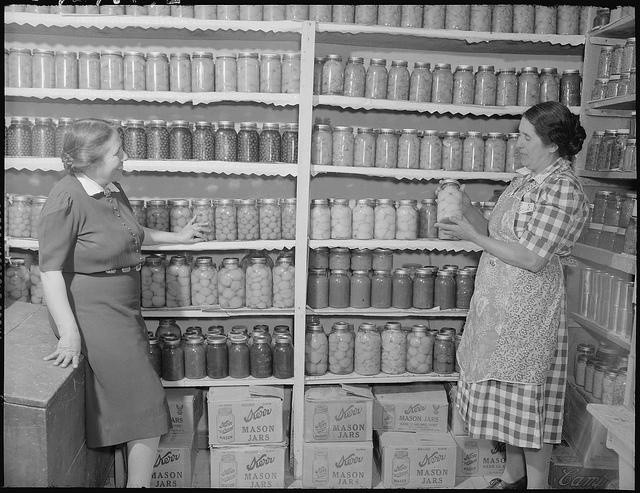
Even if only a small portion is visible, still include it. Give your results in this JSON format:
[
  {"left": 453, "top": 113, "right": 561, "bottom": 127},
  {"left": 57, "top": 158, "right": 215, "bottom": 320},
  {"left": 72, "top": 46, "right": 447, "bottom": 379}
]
[
  {"left": 36, "top": 117, "right": 212, "bottom": 488},
  {"left": 433, "top": 99, "right": 594, "bottom": 490}
]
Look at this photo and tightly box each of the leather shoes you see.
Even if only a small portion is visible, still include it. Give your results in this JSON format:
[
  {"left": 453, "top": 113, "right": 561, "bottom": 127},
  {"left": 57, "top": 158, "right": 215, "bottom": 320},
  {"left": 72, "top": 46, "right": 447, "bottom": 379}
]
[{"left": 486, "top": 474, "right": 527, "bottom": 489}]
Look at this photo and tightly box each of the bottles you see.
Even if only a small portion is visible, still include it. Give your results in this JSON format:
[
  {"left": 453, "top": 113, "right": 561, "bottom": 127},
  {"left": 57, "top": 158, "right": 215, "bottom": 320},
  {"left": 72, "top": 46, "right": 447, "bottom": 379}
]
[
  {"left": 586, "top": 128, "right": 637, "bottom": 254},
  {"left": 572, "top": 335, "right": 628, "bottom": 405},
  {"left": 5, "top": 115, "right": 297, "bottom": 163},
  {"left": 591, "top": 37, "right": 635, "bottom": 101}
]
